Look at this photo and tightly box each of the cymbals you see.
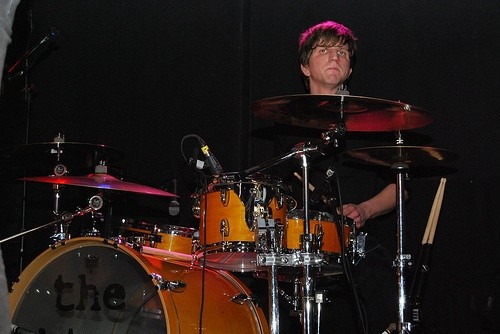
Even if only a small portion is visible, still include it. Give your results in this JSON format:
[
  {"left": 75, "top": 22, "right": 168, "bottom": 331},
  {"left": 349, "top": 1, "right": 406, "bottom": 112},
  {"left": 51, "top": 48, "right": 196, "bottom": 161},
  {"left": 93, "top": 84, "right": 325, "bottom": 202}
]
[
  {"left": 348, "top": 146, "right": 458, "bottom": 168},
  {"left": 14, "top": 177, "right": 178, "bottom": 196},
  {"left": 249, "top": 95, "right": 434, "bottom": 132},
  {"left": 24, "top": 142, "right": 110, "bottom": 148}
]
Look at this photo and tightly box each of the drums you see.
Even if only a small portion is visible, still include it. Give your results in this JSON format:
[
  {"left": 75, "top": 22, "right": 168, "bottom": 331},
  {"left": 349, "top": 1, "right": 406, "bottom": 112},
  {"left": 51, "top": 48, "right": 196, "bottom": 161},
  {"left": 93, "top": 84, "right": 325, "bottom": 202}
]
[
  {"left": 120, "top": 217, "right": 151, "bottom": 249},
  {"left": 154, "top": 222, "right": 198, "bottom": 254},
  {"left": 198, "top": 180, "right": 286, "bottom": 273},
  {"left": 288, "top": 210, "right": 358, "bottom": 276},
  {"left": 9, "top": 236, "right": 269, "bottom": 333}
]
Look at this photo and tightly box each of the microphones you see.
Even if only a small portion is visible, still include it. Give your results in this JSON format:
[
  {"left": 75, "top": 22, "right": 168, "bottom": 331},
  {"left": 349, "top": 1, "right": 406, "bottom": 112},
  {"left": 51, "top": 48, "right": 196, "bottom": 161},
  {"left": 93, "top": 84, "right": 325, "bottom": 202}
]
[
  {"left": 168, "top": 180, "right": 180, "bottom": 216},
  {"left": 198, "top": 138, "right": 222, "bottom": 174}
]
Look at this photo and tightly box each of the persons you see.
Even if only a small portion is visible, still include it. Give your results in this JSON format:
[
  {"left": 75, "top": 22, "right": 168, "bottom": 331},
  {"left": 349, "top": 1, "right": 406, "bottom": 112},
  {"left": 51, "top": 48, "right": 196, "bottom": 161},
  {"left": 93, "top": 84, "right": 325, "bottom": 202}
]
[{"left": 246, "top": 20, "right": 425, "bottom": 334}]
